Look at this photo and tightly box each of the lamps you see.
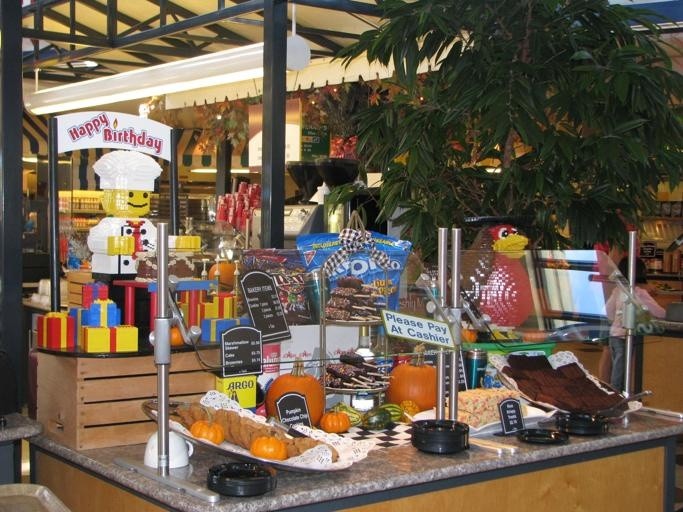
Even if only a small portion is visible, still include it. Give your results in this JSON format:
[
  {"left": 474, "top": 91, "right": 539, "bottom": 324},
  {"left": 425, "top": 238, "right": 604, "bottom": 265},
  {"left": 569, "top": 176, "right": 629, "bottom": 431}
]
[{"left": 23, "top": 4, "right": 313, "bottom": 118}]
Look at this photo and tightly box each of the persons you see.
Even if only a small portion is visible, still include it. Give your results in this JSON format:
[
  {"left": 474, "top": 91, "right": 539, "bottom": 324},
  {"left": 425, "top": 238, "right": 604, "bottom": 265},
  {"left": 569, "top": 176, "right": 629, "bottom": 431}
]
[
  {"left": 590, "top": 210, "right": 667, "bottom": 395},
  {"left": 87, "top": 150, "right": 164, "bottom": 356}
]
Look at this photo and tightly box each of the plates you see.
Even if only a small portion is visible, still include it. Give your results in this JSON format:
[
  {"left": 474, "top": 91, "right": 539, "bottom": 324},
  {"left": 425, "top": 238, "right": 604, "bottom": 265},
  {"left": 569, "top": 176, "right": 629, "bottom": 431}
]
[
  {"left": 497, "top": 366, "right": 628, "bottom": 419},
  {"left": 517, "top": 428, "right": 569, "bottom": 444},
  {"left": 412, "top": 418, "right": 469, "bottom": 455},
  {"left": 553, "top": 413, "right": 609, "bottom": 436},
  {"left": 208, "top": 461, "right": 277, "bottom": 497}
]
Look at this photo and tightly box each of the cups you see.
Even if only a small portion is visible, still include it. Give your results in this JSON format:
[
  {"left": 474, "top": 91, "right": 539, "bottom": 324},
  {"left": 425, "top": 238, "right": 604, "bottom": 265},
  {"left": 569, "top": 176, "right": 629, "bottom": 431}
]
[{"left": 143, "top": 429, "right": 193, "bottom": 468}]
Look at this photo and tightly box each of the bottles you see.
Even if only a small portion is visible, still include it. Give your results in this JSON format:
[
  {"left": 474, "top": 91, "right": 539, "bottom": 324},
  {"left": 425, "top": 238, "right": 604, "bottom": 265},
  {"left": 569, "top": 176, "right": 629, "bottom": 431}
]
[
  {"left": 466, "top": 348, "right": 486, "bottom": 390},
  {"left": 350, "top": 324, "right": 411, "bottom": 411}
]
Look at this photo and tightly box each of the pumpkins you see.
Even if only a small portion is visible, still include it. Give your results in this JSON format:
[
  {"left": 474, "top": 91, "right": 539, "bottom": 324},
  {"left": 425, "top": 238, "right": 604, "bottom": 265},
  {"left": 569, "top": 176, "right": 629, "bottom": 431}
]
[
  {"left": 387, "top": 354, "right": 437, "bottom": 422},
  {"left": 264, "top": 358, "right": 326, "bottom": 428},
  {"left": 209, "top": 263, "right": 238, "bottom": 290},
  {"left": 250, "top": 432, "right": 288, "bottom": 461},
  {"left": 524, "top": 328, "right": 545, "bottom": 342},
  {"left": 461, "top": 325, "right": 479, "bottom": 343},
  {"left": 191, "top": 412, "right": 224, "bottom": 445},
  {"left": 320, "top": 402, "right": 402, "bottom": 432},
  {"left": 171, "top": 326, "right": 184, "bottom": 347}
]
